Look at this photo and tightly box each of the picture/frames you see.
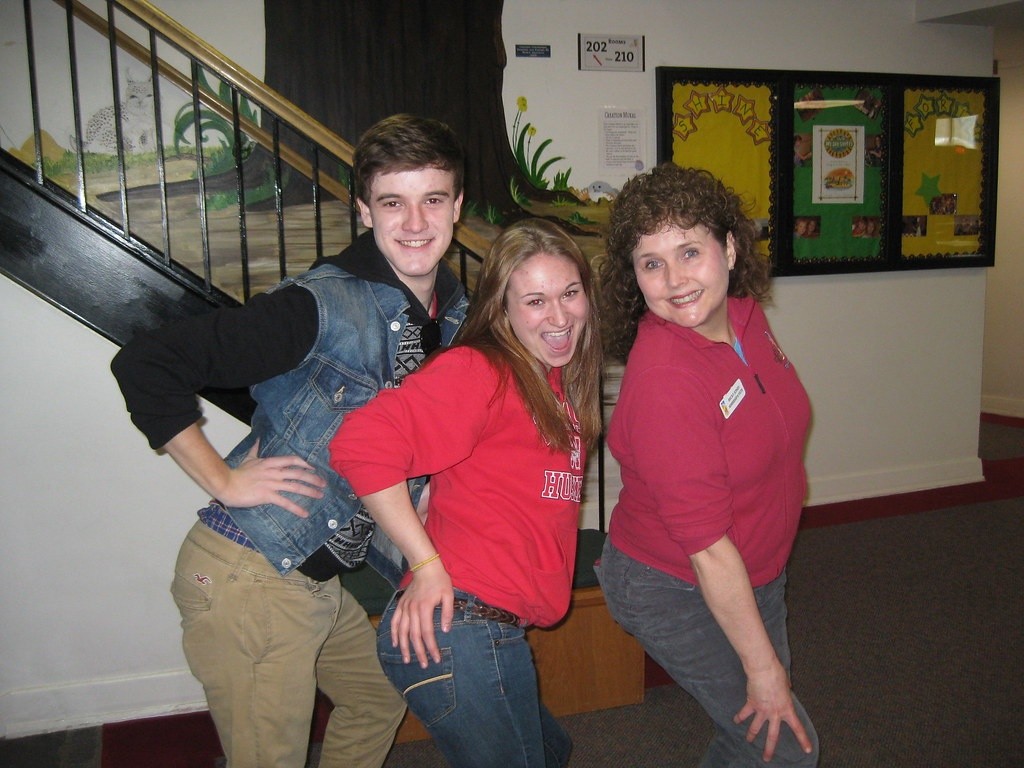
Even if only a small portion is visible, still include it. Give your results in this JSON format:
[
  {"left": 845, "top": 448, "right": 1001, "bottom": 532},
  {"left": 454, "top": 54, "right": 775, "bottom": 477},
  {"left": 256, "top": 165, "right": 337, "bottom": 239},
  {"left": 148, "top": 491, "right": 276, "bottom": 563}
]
[
  {"left": 786, "top": 70, "right": 895, "bottom": 277},
  {"left": 896, "top": 73, "right": 1001, "bottom": 272},
  {"left": 655, "top": 66, "right": 785, "bottom": 278}
]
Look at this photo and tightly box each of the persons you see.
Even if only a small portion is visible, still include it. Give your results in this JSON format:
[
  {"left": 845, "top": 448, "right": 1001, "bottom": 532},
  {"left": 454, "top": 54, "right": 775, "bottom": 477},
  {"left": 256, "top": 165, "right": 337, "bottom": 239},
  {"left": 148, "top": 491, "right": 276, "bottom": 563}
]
[
  {"left": 110, "top": 110, "right": 476, "bottom": 768},
  {"left": 589, "top": 167, "right": 823, "bottom": 767},
  {"left": 330, "top": 215, "right": 604, "bottom": 768}
]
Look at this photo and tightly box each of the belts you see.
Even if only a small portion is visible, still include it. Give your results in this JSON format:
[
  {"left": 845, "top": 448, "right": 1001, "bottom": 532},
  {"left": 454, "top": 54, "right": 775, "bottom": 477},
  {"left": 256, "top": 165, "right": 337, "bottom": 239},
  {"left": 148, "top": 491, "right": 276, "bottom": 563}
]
[{"left": 395, "top": 592, "right": 520, "bottom": 628}]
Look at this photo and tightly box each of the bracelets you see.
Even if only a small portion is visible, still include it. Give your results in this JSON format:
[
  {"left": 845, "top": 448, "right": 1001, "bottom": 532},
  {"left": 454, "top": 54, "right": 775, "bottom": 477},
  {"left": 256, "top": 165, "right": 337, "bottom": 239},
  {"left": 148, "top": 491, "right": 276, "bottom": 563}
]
[{"left": 408, "top": 552, "right": 442, "bottom": 575}]
